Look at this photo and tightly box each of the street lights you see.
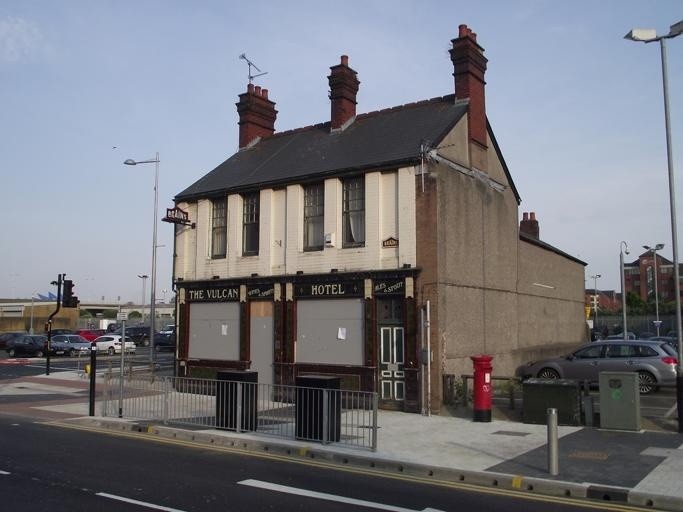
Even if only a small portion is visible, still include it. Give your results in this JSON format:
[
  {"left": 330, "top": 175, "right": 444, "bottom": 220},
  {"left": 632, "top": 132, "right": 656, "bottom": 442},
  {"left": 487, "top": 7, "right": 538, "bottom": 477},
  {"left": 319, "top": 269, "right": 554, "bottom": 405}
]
[
  {"left": 619, "top": 240, "right": 632, "bottom": 339},
  {"left": 641, "top": 243, "right": 668, "bottom": 336},
  {"left": 591, "top": 274, "right": 602, "bottom": 328},
  {"left": 136, "top": 272, "right": 149, "bottom": 323},
  {"left": 125, "top": 152, "right": 161, "bottom": 381},
  {"left": 161, "top": 289, "right": 169, "bottom": 305},
  {"left": 622, "top": 20, "right": 682, "bottom": 440}
]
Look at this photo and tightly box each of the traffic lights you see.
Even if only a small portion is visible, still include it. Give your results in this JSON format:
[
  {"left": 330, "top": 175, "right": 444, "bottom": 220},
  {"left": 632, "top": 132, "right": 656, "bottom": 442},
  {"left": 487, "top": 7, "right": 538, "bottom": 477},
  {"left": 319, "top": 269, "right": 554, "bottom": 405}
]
[{"left": 63, "top": 279, "right": 80, "bottom": 309}]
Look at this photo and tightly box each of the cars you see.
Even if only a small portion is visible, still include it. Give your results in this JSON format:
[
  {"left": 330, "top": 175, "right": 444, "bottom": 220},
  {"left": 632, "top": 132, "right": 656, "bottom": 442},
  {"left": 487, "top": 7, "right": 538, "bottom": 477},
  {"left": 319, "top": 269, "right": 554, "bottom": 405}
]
[
  {"left": 524, "top": 325, "right": 679, "bottom": 395},
  {"left": 0, "top": 324, "right": 176, "bottom": 358}
]
